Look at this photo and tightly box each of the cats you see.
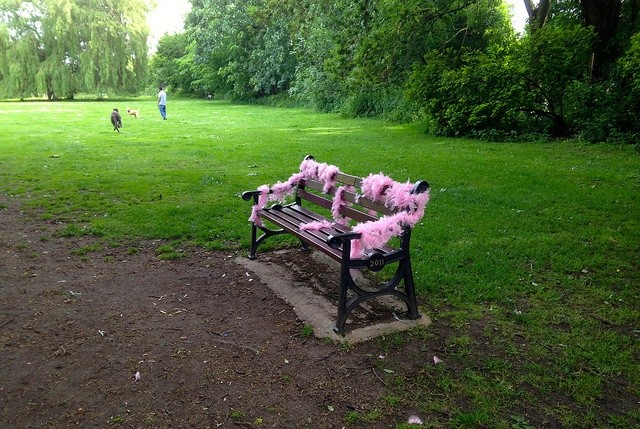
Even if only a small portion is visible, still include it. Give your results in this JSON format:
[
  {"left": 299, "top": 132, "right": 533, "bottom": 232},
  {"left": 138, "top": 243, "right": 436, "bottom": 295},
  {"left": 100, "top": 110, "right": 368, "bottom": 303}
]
[{"left": 110, "top": 108, "right": 123, "bottom": 134}]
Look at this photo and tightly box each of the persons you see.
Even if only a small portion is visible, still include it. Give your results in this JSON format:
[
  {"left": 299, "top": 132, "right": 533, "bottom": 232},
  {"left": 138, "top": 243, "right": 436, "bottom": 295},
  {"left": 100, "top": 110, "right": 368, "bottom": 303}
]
[{"left": 157, "top": 87, "right": 166, "bottom": 120}]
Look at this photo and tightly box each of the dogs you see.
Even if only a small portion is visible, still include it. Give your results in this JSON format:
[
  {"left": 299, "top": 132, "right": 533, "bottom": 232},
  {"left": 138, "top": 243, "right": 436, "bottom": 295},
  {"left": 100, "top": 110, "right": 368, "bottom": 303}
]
[{"left": 126, "top": 107, "right": 140, "bottom": 119}]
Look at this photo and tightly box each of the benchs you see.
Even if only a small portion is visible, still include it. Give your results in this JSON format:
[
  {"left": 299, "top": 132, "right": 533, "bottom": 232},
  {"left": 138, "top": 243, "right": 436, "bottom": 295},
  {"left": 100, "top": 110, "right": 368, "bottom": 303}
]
[{"left": 240, "top": 152, "right": 431, "bottom": 331}]
